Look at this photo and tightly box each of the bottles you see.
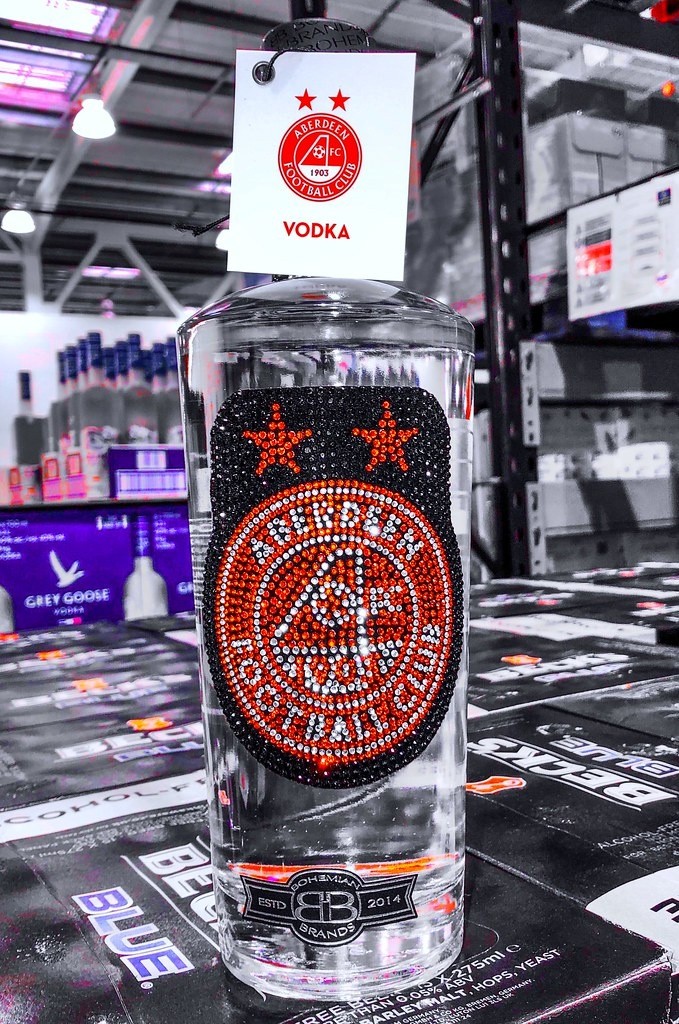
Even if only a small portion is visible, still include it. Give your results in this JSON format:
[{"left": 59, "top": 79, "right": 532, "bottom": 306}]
[
  {"left": 14, "top": 371, "right": 45, "bottom": 466},
  {"left": 122, "top": 512, "right": 169, "bottom": 622},
  {"left": 178, "top": 18, "right": 477, "bottom": 1005},
  {"left": 47, "top": 332, "right": 183, "bottom": 453},
  {"left": 0, "top": 584, "right": 14, "bottom": 636}
]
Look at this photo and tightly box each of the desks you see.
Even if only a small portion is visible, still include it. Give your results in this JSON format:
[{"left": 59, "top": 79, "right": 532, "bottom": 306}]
[{"left": 0, "top": 493, "right": 195, "bottom": 630}]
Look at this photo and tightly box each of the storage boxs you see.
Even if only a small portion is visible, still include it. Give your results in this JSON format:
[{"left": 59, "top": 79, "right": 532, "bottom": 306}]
[
  {"left": 405, "top": 155, "right": 491, "bottom": 323},
  {"left": 9, "top": 465, "right": 43, "bottom": 505},
  {"left": 527, "top": 228, "right": 571, "bottom": 305},
  {"left": 0, "top": 564, "right": 679, "bottom": 1024},
  {"left": 628, "top": 97, "right": 679, "bottom": 132},
  {"left": 624, "top": 120, "right": 679, "bottom": 184},
  {"left": 65, "top": 448, "right": 110, "bottom": 498},
  {"left": 107, "top": 443, "right": 190, "bottom": 502},
  {"left": 526, "top": 77, "right": 626, "bottom": 126},
  {"left": 41, "top": 452, "right": 68, "bottom": 500},
  {"left": 412, "top": 53, "right": 479, "bottom": 165},
  {"left": 523, "top": 110, "right": 627, "bottom": 225}
]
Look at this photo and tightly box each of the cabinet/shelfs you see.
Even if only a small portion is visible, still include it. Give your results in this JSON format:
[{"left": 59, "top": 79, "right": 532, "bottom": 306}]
[{"left": 420, "top": 0, "right": 679, "bottom": 582}]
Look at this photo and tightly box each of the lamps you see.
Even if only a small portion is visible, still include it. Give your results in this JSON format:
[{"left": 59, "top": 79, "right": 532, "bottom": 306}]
[
  {"left": 71, "top": 73, "right": 116, "bottom": 139},
  {"left": 1, "top": 191, "right": 36, "bottom": 234}
]
